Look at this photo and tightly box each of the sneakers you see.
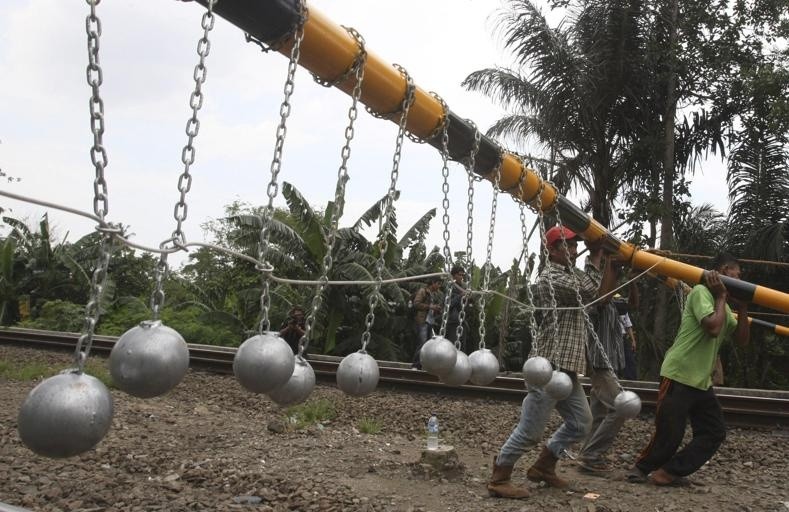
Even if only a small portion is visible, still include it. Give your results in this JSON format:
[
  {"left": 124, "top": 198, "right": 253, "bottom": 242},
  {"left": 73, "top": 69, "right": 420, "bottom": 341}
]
[
  {"left": 624, "top": 467, "right": 686, "bottom": 486},
  {"left": 579, "top": 460, "right": 614, "bottom": 473}
]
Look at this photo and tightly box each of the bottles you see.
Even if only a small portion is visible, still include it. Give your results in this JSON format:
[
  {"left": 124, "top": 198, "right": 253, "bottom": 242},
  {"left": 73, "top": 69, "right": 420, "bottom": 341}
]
[{"left": 426, "top": 413, "right": 440, "bottom": 451}]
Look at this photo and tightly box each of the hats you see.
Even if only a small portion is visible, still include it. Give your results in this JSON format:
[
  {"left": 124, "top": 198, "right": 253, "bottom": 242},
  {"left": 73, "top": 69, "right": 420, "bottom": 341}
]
[{"left": 545, "top": 226, "right": 583, "bottom": 245}]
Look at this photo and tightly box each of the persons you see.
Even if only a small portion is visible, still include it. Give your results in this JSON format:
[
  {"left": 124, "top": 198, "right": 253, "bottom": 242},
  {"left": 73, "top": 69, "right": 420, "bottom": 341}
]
[
  {"left": 411, "top": 266, "right": 470, "bottom": 371},
  {"left": 279, "top": 311, "right": 307, "bottom": 355},
  {"left": 612, "top": 293, "right": 637, "bottom": 379},
  {"left": 637, "top": 252, "right": 750, "bottom": 485},
  {"left": 487, "top": 227, "right": 603, "bottom": 499},
  {"left": 576, "top": 249, "right": 641, "bottom": 473}
]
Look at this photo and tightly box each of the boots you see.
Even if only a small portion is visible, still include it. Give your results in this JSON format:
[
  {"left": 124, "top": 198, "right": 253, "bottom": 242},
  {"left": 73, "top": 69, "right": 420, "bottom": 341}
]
[
  {"left": 526, "top": 448, "right": 569, "bottom": 489},
  {"left": 486, "top": 454, "right": 531, "bottom": 499}
]
